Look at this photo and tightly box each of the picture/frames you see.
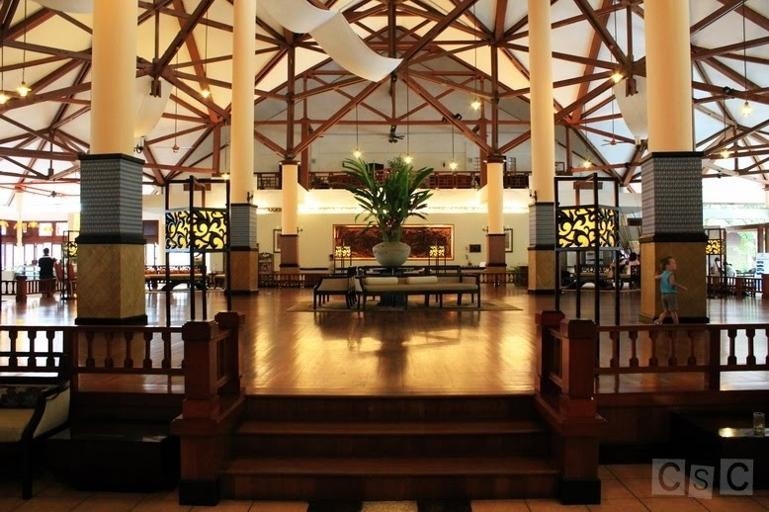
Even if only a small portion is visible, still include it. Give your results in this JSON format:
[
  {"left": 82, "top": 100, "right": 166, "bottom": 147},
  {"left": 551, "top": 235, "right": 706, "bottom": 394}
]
[
  {"left": 332, "top": 222, "right": 456, "bottom": 261},
  {"left": 273, "top": 229, "right": 281, "bottom": 254},
  {"left": 504, "top": 227, "right": 513, "bottom": 253}
]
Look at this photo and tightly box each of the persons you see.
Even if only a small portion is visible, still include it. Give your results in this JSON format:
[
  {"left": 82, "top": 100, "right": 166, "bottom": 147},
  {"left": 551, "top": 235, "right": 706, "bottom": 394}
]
[
  {"left": 711, "top": 257, "right": 736, "bottom": 286},
  {"left": 38, "top": 248, "right": 57, "bottom": 279},
  {"left": 625, "top": 252, "right": 640, "bottom": 275},
  {"left": 653, "top": 256, "right": 688, "bottom": 325}
]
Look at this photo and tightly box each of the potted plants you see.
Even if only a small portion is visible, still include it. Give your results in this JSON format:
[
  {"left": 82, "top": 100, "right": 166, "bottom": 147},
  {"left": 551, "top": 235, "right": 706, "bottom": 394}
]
[{"left": 331, "top": 154, "right": 436, "bottom": 267}]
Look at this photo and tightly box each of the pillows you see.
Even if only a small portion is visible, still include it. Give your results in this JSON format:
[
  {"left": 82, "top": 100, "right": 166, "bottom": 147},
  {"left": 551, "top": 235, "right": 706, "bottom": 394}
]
[
  {"left": 365, "top": 276, "right": 399, "bottom": 286},
  {"left": 406, "top": 275, "right": 439, "bottom": 284}
]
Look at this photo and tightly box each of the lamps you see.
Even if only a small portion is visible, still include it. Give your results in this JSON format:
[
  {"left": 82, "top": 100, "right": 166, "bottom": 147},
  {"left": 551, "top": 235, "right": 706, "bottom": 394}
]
[
  {"left": 164, "top": 175, "right": 233, "bottom": 321},
  {"left": 151, "top": 0, "right": 627, "bottom": 174},
  {"left": 703, "top": 227, "right": 728, "bottom": 301},
  {"left": 61, "top": 229, "right": 80, "bottom": 303},
  {"left": 428, "top": 245, "right": 447, "bottom": 273},
  {"left": 720, "top": 90, "right": 730, "bottom": 161},
  {"left": 551, "top": 172, "right": 622, "bottom": 326},
  {"left": 333, "top": 245, "right": 352, "bottom": 273},
  {"left": 16, "top": 0, "right": 33, "bottom": 99},
  {"left": 737, "top": 1, "right": 756, "bottom": 120},
  {"left": 1, "top": 0, "right": 11, "bottom": 106}
]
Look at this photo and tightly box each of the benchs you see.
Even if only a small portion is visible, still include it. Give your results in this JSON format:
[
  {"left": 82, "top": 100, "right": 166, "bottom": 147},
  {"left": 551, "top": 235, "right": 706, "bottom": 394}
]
[
  {"left": 357, "top": 266, "right": 487, "bottom": 315},
  {"left": 143, "top": 264, "right": 208, "bottom": 291}
]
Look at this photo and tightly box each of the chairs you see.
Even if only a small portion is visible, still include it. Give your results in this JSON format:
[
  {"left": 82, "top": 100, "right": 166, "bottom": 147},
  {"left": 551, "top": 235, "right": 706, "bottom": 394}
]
[{"left": 312, "top": 265, "right": 357, "bottom": 306}]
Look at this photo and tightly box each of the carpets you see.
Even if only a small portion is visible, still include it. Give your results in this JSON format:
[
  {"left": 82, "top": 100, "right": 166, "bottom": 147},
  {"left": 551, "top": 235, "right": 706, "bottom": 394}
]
[{"left": 286, "top": 300, "right": 524, "bottom": 314}]
